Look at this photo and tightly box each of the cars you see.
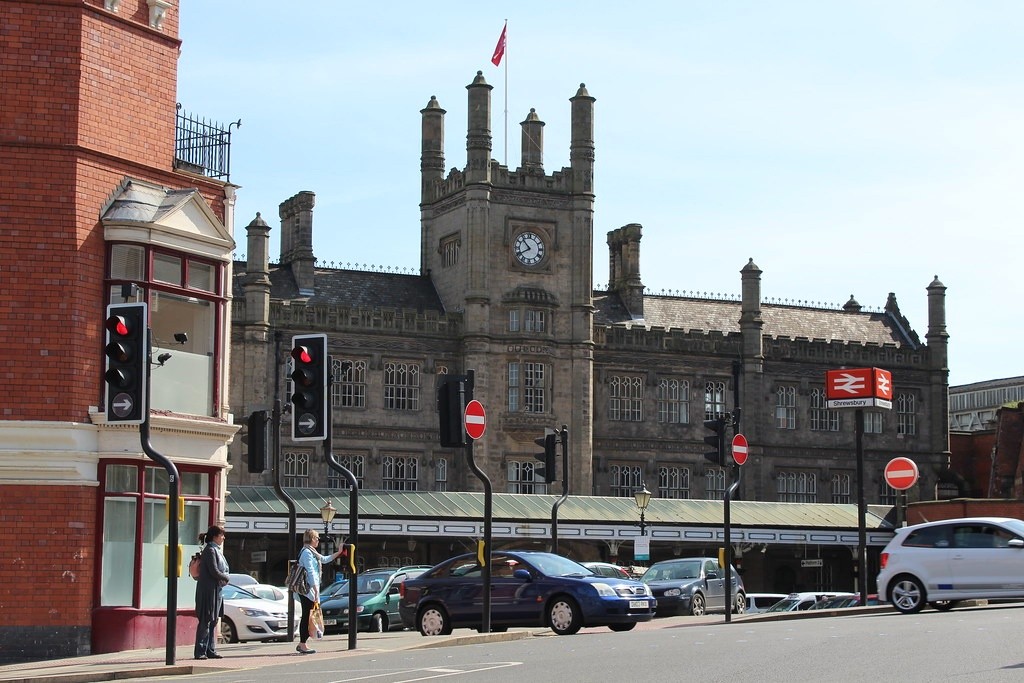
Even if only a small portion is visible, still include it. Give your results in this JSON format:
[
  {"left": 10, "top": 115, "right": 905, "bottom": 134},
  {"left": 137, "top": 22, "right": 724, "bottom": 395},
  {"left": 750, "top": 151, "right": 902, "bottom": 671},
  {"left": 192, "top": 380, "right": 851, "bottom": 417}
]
[
  {"left": 317, "top": 579, "right": 385, "bottom": 604},
  {"left": 216, "top": 573, "right": 303, "bottom": 645},
  {"left": 581, "top": 562, "right": 633, "bottom": 581},
  {"left": 398, "top": 550, "right": 658, "bottom": 636},
  {"left": 744, "top": 591, "right": 886, "bottom": 615},
  {"left": 638, "top": 556, "right": 747, "bottom": 617}
]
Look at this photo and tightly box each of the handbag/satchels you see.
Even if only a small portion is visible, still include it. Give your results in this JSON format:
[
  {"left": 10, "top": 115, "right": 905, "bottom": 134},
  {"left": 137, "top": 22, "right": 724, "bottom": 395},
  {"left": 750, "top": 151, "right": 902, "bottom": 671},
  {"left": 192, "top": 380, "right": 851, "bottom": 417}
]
[
  {"left": 308, "top": 602, "right": 325, "bottom": 641},
  {"left": 286, "top": 549, "right": 318, "bottom": 595}
]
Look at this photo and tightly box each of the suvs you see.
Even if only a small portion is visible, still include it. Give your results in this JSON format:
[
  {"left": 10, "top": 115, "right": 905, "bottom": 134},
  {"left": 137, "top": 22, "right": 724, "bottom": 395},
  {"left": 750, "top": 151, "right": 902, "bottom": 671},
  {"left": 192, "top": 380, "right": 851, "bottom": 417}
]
[
  {"left": 875, "top": 516, "right": 1024, "bottom": 613},
  {"left": 320, "top": 565, "right": 437, "bottom": 635}
]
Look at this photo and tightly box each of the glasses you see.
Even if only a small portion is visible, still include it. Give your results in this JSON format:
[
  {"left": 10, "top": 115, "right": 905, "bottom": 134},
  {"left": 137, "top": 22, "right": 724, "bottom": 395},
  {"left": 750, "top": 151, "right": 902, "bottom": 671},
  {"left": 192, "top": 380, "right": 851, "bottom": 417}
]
[{"left": 314, "top": 536, "right": 320, "bottom": 540}]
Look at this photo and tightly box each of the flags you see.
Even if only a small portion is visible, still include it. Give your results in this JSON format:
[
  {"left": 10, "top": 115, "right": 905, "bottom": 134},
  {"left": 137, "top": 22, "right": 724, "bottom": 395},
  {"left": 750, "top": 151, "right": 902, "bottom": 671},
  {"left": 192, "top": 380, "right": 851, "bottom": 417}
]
[{"left": 491, "top": 25, "right": 506, "bottom": 67}]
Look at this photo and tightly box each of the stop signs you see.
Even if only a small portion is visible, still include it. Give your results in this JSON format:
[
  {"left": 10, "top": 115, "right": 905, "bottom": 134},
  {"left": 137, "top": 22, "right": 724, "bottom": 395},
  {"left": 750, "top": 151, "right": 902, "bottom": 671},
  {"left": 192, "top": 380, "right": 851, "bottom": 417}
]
[
  {"left": 732, "top": 434, "right": 749, "bottom": 465},
  {"left": 464, "top": 400, "right": 487, "bottom": 440},
  {"left": 884, "top": 457, "right": 918, "bottom": 490}
]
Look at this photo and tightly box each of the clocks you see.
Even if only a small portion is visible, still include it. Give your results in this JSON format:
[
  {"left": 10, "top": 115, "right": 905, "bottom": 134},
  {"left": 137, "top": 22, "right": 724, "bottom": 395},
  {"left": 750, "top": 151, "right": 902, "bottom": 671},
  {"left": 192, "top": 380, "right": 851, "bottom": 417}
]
[{"left": 511, "top": 225, "right": 552, "bottom": 271}]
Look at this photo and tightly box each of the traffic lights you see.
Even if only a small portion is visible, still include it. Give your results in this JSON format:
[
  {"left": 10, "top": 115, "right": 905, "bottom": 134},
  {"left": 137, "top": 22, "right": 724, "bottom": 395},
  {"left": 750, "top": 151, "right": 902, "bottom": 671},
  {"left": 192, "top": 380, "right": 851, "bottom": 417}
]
[
  {"left": 534, "top": 427, "right": 559, "bottom": 484},
  {"left": 702, "top": 417, "right": 729, "bottom": 466},
  {"left": 104, "top": 303, "right": 147, "bottom": 421},
  {"left": 288, "top": 334, "right": 328, "bottom": 440},
  {"left": 242, "top": 411, "right": 266, "bottom": 472}
]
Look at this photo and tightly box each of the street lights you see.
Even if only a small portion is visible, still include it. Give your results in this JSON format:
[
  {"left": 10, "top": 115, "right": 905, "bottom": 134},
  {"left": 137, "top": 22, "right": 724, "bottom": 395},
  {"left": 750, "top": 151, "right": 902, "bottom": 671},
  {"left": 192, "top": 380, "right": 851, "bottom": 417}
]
[{"left": 633, "top": 480, "right": 654, "bottom": 566}]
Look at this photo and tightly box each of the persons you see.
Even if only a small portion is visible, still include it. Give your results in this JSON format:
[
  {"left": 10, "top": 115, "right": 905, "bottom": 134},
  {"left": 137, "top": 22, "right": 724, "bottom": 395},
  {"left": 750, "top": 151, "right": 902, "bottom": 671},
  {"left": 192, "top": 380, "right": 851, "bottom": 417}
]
[
  {"left": 195, "top": 526, "right": 229, "bottom": 659},
  {"left": 995, "top": 530, "right": 1010, "bottom": 547},
  {"left": 512, "top": 563, "right": 527, "bottom": 571},
  {"left": 296, "top": 529, "right": 346, "bottom": 653}
]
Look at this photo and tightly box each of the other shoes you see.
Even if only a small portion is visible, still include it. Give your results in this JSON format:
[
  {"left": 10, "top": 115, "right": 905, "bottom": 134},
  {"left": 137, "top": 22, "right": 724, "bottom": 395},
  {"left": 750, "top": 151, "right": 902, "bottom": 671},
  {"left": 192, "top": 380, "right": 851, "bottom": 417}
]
[
  {"left": 208, "top": 653, "right": 223, "bottom": 659},
  {"left": 296, "top": 645, "right": 316, "bottom": 654},
  {"left": 195, "top": 654, "right": 208, "bottom": 659}
]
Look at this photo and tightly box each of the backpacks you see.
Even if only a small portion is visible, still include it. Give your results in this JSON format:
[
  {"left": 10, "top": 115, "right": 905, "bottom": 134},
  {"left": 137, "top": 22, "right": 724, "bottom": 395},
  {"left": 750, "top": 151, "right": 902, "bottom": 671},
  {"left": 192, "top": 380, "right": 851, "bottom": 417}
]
[{"left": 188, "top": 546, "right": 219, "bottom": 580}]
